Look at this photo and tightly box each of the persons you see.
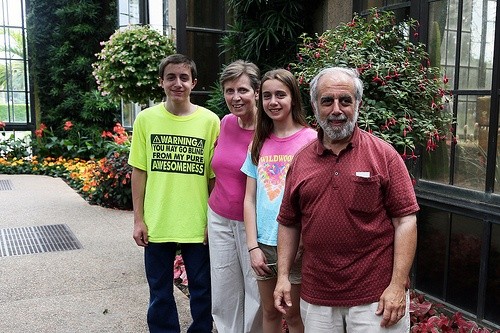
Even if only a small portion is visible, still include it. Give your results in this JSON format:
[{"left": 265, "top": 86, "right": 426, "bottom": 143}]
[
  {"left": 272, "top": 66, "right": 422, "bottom": 332},
  {"left": 126, "top": 53, "right": 216, "bottom": 333},
  {"left": 204, "top": 59, "right": 265, "bottom": 333},
  {"left": 239, "top": 66, "right": 320, "bottom": 332}
]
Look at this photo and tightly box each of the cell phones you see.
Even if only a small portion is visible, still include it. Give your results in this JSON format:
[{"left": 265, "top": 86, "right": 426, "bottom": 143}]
[{"left": 267, "top": 263, "right": 278, "bottom": 275}]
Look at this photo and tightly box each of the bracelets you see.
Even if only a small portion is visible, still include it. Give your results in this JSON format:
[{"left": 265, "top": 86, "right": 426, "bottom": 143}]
[{"left": 248, "top": 246, "right": 260, "bottom": 251}]
